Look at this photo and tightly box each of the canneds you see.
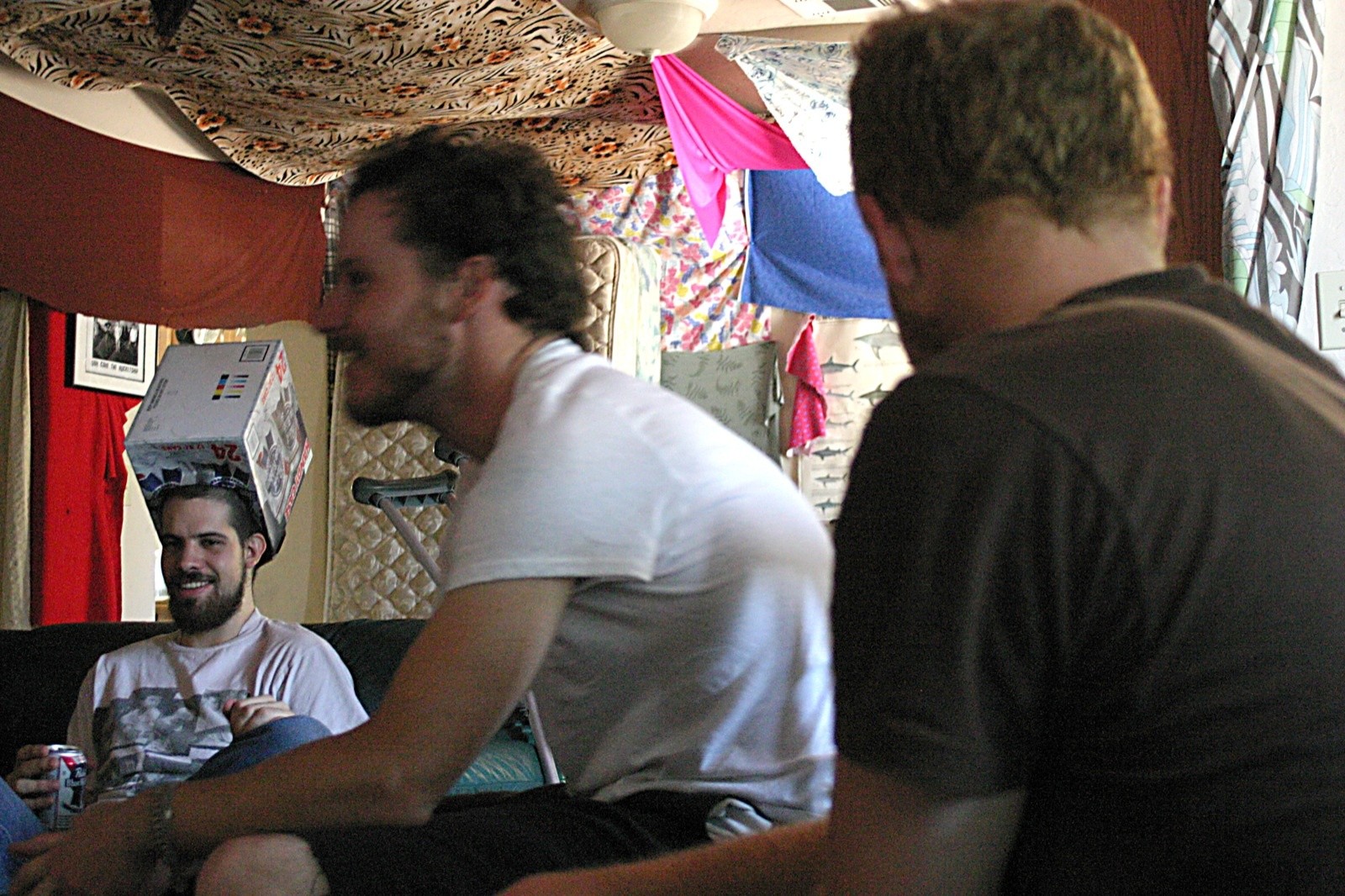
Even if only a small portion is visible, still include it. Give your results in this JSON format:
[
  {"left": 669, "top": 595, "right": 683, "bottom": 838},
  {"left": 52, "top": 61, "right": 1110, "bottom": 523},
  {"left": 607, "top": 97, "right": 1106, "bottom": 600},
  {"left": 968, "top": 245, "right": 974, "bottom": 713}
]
[{"left": 39, "top": 744, "right": 87, "bottom": 830}]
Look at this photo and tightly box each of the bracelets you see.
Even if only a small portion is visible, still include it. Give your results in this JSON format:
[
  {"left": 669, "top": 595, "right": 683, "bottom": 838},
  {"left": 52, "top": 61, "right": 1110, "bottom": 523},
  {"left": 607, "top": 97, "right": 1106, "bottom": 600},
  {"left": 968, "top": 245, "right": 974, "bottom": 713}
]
[{"left": 143, "top": 780, "right": 183, "bottom": 864}]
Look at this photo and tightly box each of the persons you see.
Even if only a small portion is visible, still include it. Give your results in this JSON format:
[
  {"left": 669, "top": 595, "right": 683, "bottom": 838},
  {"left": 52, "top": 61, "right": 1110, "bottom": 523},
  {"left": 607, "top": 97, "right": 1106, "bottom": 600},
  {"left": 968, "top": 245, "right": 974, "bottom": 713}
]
[
  {"left": 1, "top": 481, "right": 371, "bottom": 896},
  {"left": 0, "top": 120, "right": 834, "bottom": 896},
  {"left": 501, "top": 2, "right": 1345, "bottom": 896}
]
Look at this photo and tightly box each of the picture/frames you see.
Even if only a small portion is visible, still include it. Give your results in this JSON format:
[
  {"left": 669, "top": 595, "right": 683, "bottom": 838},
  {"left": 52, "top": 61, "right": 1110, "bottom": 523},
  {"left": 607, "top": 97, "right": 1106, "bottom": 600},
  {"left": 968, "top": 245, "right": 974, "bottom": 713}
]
[{"left": 64, "top": 314, "right": 159, "bottom": 400}]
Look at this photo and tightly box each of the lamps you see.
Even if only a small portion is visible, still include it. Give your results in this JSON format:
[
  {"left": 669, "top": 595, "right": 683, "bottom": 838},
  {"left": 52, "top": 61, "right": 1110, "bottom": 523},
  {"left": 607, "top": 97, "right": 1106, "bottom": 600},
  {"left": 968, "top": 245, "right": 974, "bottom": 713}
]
[{"left": 586, "top": 1, "right": 720, "bottom": 62}]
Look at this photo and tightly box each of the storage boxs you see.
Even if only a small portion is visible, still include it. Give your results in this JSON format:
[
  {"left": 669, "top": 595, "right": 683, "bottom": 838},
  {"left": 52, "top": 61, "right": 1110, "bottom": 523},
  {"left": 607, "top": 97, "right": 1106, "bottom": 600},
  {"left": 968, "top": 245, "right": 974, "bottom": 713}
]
[{"left": 126, "top": 337, "right": 312, "bottom": 558}]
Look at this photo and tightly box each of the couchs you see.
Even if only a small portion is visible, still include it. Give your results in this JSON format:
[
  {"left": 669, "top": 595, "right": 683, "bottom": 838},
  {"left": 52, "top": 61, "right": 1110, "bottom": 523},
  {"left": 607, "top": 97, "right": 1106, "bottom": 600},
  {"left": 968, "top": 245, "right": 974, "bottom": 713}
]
[{"left": 0, "top": 618, "right": 567, "bottom": 797}]
